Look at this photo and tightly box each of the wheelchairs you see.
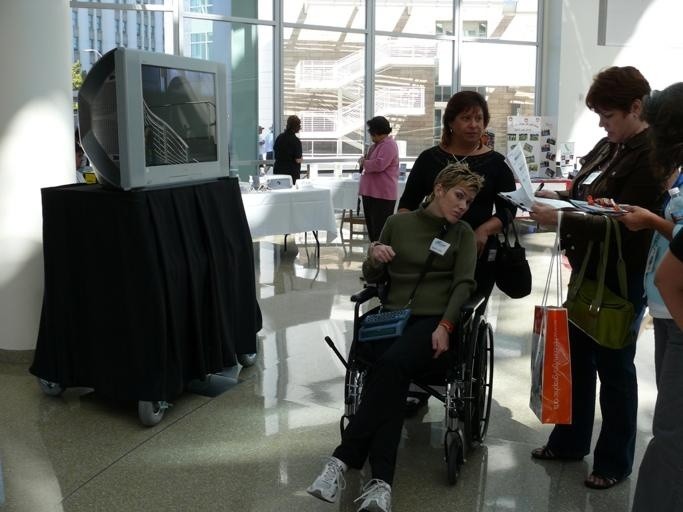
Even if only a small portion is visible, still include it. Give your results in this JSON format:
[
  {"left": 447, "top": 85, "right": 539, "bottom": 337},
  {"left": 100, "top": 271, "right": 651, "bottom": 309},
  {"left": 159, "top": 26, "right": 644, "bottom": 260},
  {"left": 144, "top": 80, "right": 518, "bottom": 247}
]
[{"left": 325, "top": 234, "right": 504, "bottom": 488}]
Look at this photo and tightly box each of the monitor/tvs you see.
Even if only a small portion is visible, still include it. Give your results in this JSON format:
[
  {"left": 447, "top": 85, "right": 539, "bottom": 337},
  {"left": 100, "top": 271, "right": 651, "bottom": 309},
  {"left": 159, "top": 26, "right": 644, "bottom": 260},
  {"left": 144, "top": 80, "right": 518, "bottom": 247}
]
[{"left": 76, "top": 45, "right": 231, "bottom": 192}]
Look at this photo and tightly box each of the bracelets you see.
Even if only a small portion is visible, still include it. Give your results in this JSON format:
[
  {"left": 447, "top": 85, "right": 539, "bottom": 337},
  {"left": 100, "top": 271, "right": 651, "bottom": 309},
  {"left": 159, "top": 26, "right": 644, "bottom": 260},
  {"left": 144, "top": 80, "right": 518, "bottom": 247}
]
[{"left": 439, "top": 320, "right": 454, "bottom": 335}]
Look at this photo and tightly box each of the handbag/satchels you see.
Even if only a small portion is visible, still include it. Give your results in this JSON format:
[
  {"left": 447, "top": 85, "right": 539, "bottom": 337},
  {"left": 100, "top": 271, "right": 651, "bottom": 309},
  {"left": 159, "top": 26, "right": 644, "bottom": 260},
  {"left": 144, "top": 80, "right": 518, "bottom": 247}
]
[
  {"left": 562, "top": 214, "right": 634, "bottom": 349},
  {"left": 494, "top": 212, "right": 532, "bottom": 299},
  {"left": 357, "top": 306, "right": 411, "bottom": 343},
  {"left": 529, "top": 212, "right": 573, "bottom": 425}
]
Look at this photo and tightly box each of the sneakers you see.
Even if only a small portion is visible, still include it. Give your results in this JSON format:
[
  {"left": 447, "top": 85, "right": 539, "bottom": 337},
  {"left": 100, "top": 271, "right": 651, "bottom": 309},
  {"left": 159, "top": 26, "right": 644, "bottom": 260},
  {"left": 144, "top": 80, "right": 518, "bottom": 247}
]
[
  {"left": 353, "top": 478, "right": 392, "bottom": 511},
  {"left": 306, "top": 457, "right": 349, "bottom": 504}
]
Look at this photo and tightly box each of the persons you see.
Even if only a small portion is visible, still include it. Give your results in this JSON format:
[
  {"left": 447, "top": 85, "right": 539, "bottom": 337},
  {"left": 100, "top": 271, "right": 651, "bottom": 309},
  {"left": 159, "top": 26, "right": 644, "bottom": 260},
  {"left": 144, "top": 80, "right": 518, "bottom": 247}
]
[
  {"left": 258, "top": 125, "right": 266, "bottom": 169},
  {"left": 271, "top": 115, "right": 306, "bottom": 184},
  {"left": 591, "top": 80, "right": 683, "bottom": 510},
  {"left": 527, "top": 62, "right": 662, "bottom": 491},
  {"left": 650, "top": 224, "right": 682, "bottom": 332},
  {"left": 357, "top": 115, "right": 401, "bottom": 243},
  {"left": 396, "top": 89, "right": 519, "bottom": 421},
  {"left": 276, "top": 238, "right": 299, "bottom": 297},
  {"left": 304, "top": 158, "right": 490, "bottom": 512}
]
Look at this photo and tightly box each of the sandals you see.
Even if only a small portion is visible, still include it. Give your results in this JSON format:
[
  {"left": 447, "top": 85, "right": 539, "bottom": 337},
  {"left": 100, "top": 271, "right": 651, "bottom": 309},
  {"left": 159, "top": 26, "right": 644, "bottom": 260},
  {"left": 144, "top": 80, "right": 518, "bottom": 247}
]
[
  {"left": 531, "top": 446, "right": 583, "bottom": 461},
  {"left": 584, "top": 471, "right": 632, "bottom": 489}
]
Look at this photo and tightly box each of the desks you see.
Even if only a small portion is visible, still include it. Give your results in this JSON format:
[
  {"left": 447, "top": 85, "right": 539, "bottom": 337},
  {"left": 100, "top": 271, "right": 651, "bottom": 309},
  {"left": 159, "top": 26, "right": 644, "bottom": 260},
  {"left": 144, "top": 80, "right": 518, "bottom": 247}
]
[{"left": 239, "top": 172, "right": 408, "bottom": 262}]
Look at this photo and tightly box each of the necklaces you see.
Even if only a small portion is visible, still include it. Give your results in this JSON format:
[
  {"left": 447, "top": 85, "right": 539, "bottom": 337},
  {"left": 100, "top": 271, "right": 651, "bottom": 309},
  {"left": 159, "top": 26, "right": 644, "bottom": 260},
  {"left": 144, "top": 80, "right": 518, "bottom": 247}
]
[{"left": 448, "top": 144, "right": 480, "bottom": 164}]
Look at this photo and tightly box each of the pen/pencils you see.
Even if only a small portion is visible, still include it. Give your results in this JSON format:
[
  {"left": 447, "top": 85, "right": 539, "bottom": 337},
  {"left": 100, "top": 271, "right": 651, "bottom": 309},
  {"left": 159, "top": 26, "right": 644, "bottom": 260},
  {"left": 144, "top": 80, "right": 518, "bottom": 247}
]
[{"left": 535, "top": 182, "right": 544, "bottom": 196}]
